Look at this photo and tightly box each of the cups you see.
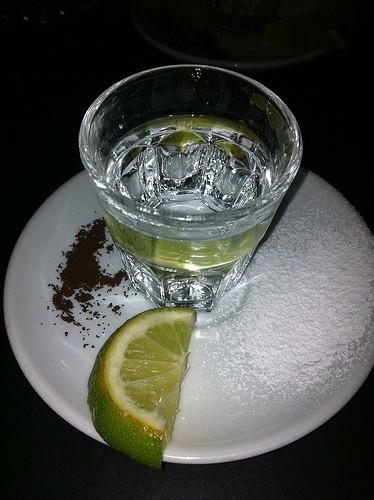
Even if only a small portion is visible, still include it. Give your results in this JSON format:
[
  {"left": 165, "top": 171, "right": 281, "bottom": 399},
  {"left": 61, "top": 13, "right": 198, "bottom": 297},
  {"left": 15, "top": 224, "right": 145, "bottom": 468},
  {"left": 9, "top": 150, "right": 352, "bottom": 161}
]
[{"left": 80, "top": 64, "right": 305, "bottom": 311}]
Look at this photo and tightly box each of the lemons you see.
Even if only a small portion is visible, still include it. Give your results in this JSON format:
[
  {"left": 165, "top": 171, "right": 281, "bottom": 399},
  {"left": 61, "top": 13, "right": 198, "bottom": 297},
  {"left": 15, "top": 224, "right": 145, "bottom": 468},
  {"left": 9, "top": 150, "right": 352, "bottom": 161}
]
[{"left": 87, "top": 307, "right": 196, "bottom": 470}]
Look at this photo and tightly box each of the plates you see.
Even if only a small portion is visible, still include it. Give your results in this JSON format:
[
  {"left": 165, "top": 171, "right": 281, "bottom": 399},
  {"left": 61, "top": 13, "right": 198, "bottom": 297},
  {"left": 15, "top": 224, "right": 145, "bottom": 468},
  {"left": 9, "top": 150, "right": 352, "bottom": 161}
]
[{"left": 4, "top": 155, "right": 374, "bottom": 469}]
